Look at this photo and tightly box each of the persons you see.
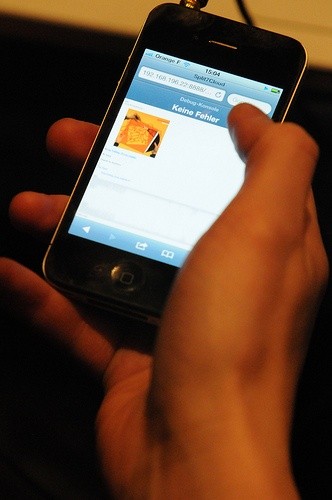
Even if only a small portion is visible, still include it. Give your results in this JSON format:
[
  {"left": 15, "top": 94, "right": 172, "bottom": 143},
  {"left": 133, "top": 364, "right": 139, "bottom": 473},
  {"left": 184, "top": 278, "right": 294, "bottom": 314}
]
[{"left": 1, "top": 101, "right": 330, "bottom": 498}]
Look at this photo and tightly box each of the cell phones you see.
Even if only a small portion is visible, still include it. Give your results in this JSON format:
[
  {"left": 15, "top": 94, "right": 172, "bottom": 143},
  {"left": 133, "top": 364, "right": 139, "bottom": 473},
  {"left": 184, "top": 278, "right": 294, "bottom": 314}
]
[{"left": 43, "top": 3, "right": 307, "bottom": 327}]
